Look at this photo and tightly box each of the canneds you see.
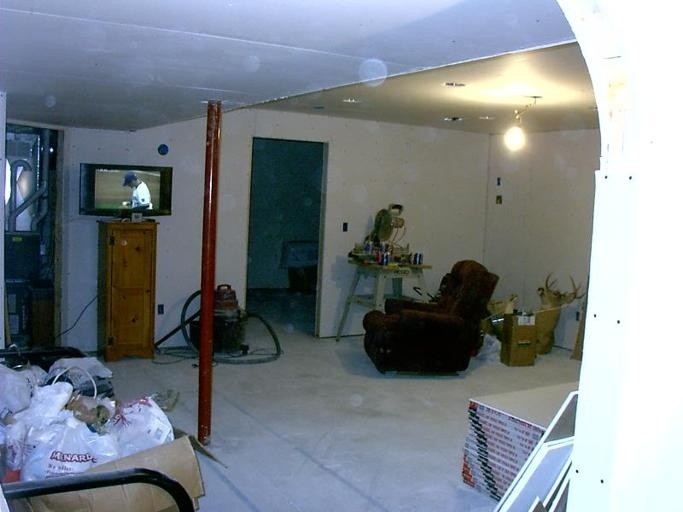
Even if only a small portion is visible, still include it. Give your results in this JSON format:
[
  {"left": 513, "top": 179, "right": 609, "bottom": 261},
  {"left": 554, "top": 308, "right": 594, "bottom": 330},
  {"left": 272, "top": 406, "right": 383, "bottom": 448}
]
[
  {"left": 365, "top": 241, "right": 373, "bottom": 255},
  {"left": 413, "top": 253, "right": 423, "bottom": 265},
  {"left": 381, "top": 252, "right": 390, "bottom": 266}
]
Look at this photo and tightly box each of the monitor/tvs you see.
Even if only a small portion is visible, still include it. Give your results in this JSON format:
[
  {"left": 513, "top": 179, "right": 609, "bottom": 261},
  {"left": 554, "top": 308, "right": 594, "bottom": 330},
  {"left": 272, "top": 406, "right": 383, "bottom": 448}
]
[{"left": 79, "top": 163, "right": 172, "bottom": 222}]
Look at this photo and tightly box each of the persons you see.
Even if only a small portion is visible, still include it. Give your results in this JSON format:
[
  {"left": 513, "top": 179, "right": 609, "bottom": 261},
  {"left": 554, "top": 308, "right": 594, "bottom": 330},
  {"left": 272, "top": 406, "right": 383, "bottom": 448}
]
[{"left": 122, "top": 173, "right": 152, "bottom": 210}]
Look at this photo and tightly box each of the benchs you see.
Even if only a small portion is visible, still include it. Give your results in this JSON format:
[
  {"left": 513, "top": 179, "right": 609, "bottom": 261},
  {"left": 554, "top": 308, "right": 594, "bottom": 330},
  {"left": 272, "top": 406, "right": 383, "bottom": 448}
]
[{"left": 0, "top": 345, "right": 196, "bottom": 512}]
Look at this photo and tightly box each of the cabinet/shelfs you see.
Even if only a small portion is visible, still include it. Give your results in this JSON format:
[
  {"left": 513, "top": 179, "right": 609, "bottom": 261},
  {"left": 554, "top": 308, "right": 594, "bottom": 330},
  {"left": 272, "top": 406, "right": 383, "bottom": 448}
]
[{"left": 95, "top": 219, "right": 161, "bottom": 363}]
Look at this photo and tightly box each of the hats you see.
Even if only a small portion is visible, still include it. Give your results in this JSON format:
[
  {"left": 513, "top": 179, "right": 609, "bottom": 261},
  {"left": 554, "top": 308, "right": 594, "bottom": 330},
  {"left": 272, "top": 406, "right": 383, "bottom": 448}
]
[{"left": 122, "top": 172, "right": 139, "bottom": 187}]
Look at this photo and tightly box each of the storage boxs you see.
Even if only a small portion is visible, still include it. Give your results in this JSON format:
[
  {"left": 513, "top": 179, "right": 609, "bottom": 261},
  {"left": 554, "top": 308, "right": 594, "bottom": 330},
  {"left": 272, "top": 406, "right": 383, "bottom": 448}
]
[
  {"left": 0, "top": 425, "right": 225, "bottom": 512},
  {"left": 500, "top": 312, "right": 537, "bottom": 368}
]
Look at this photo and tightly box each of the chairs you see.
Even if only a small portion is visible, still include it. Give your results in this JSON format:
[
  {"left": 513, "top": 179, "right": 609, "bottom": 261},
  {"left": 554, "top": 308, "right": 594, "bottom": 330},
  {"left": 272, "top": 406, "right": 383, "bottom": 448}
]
[{"left": 361, "top": 258, "right": 500, "bottom": 377}]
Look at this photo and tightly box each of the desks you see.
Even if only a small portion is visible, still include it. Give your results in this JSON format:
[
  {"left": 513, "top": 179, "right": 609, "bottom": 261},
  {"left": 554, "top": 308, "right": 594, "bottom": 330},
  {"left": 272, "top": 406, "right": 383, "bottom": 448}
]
[{"left": 336, "top": 255, "right": 433, "bottom": 342}]
